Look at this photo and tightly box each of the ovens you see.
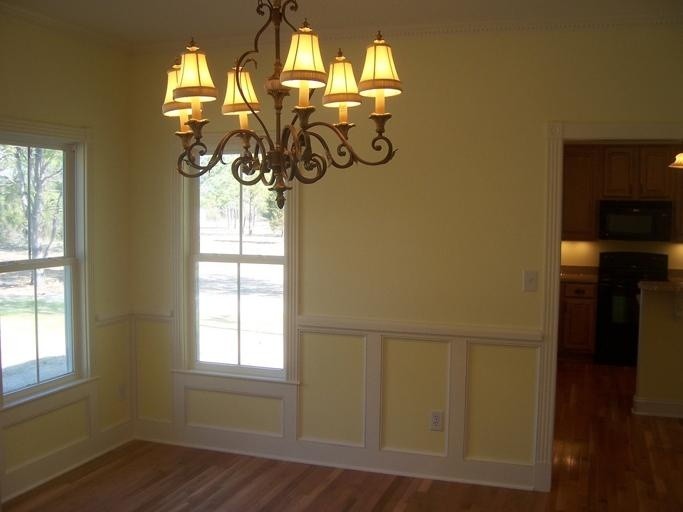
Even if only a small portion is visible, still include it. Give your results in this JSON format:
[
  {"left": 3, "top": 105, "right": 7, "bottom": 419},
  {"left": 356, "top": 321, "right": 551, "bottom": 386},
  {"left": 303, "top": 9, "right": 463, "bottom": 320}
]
[{"left": 598, "top": 283, "right": 642, "bottom": 367}]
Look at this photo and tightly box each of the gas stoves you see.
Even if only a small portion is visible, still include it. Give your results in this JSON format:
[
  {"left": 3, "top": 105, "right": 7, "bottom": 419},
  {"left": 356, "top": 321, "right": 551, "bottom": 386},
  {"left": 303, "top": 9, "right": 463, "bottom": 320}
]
[{"left": 598, "top": 270, "right": 670, "bottom": 284}]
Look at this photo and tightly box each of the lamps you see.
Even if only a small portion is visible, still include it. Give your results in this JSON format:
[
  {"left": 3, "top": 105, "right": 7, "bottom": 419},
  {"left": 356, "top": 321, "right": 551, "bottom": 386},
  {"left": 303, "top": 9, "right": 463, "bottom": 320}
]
[{"left": 160, "top": 0, "right": 402, "bottom": 212}]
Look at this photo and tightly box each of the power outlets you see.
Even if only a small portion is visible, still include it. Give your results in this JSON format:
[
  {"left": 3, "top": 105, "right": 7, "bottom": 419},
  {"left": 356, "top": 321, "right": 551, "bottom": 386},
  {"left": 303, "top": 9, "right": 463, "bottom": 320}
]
[{"left": 427, "top": 408, "right": 442, "bottom": 431}]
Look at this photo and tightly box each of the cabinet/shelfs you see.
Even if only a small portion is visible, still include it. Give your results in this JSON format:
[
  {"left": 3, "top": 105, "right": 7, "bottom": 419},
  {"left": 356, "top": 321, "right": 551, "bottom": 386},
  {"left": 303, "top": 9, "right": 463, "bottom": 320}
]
[
  {"left": 561, "top": 145, "right": 599, "bottom": 243},
  {"left": 669, "top": 143, "right": 682, "bottom": 243},
  {"left": 598, "top": 146, "right": 672, "bottom": 199},
  {"left": 559, "top": 279, "right": 595, "bottom": 354}
]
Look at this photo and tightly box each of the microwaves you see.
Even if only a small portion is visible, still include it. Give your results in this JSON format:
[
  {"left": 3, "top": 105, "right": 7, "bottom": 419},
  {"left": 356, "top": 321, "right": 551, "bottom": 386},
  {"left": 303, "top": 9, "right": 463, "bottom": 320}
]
[{"left": 599, "top": 200, "right": 674, "bottom": 242}]
